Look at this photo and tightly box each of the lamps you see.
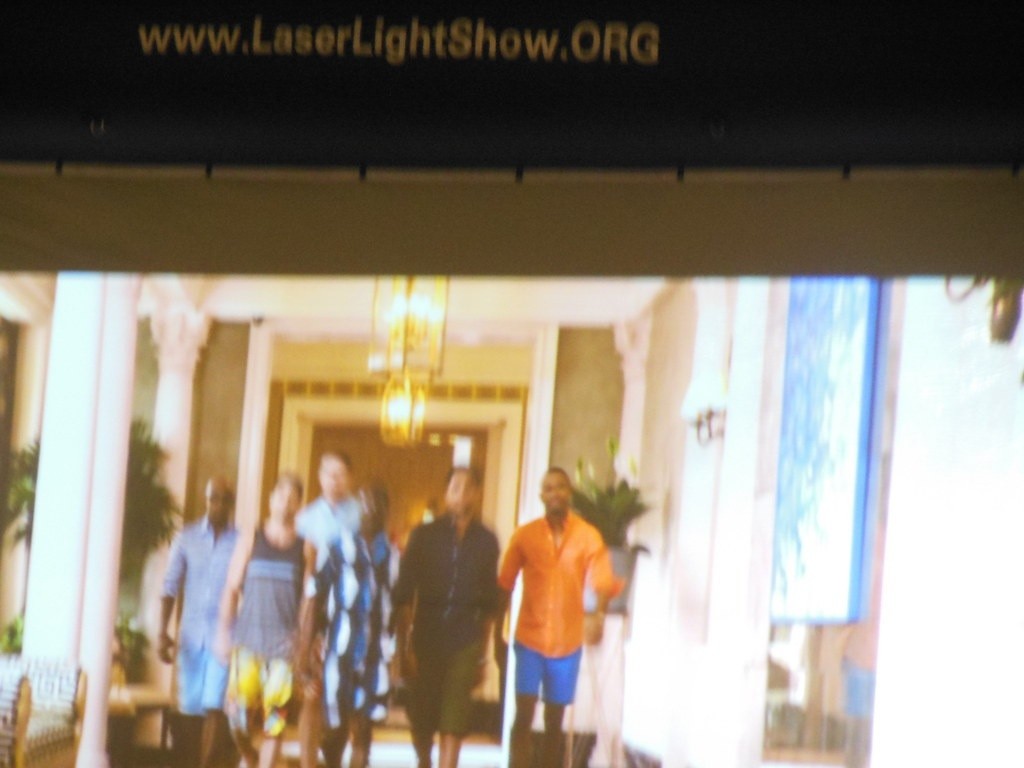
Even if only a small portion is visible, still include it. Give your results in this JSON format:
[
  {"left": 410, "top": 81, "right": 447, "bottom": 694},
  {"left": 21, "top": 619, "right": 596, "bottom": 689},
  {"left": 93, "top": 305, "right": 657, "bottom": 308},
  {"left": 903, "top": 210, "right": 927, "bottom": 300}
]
[{"left": 365, "top": 277, "right": 455, "bottom": 450}]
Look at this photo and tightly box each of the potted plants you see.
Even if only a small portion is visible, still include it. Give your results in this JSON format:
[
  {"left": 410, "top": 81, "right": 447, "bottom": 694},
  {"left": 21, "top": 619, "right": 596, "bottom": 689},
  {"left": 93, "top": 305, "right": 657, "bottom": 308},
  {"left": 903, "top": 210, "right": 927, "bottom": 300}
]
[{"left": 568, "top": 438, "right": 649, "bottom": 616}]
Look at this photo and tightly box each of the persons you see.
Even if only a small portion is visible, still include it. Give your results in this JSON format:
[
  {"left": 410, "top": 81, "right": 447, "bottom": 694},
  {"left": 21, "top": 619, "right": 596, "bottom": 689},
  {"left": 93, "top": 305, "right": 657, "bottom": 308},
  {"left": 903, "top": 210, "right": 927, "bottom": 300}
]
[{"left": 155, "top": 451, "right": 626, "bottom": 768}]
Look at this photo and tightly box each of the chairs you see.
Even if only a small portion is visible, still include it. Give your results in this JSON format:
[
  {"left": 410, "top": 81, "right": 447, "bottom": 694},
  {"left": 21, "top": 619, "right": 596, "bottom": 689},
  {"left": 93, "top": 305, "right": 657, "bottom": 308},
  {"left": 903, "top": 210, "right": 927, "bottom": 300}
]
[{"left": 2, "top": 650, "right": 88, "bottom": 768}]
